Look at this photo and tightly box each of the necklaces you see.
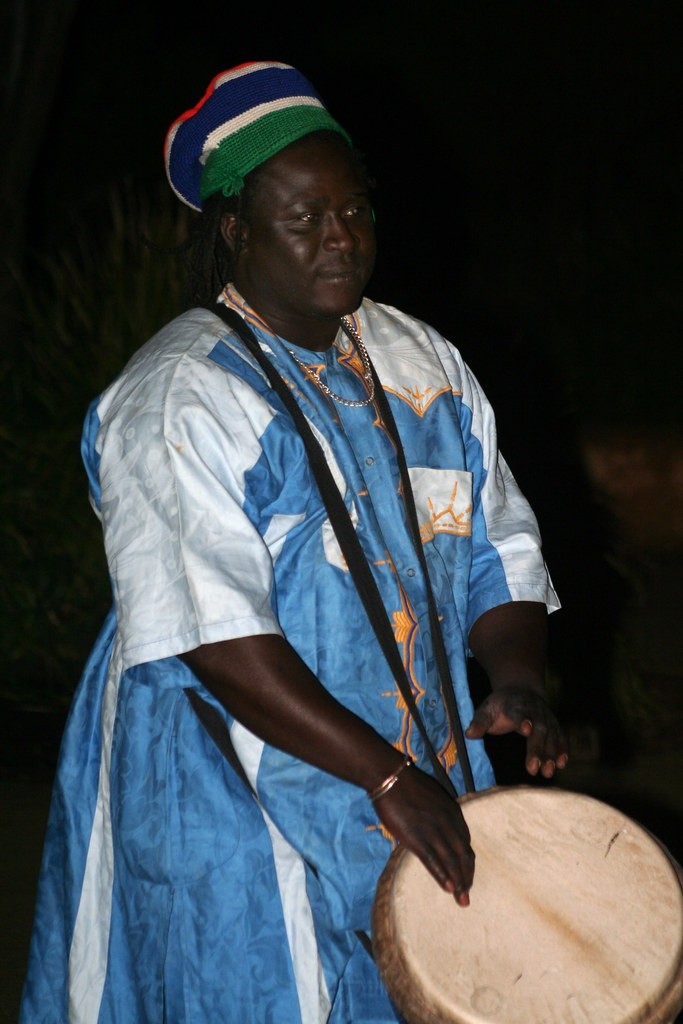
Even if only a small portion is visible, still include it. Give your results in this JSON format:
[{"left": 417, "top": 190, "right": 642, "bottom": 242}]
[{"left": 221, "top": 269, "right": 376, "bottom": 407}]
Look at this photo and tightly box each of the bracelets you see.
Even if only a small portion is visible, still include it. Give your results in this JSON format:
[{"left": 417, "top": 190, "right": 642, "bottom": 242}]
[{"left": 367, "top": 747, "right": 413, "bottom": 805}]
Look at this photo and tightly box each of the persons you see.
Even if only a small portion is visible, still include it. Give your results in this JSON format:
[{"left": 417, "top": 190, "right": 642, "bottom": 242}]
[{"left": 17, "top": 57, "right": 585, "bottom": 1024}]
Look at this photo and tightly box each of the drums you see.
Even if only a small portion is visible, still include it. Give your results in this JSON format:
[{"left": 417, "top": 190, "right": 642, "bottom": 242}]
[{"left": 367, "top": 784, "right": 683, "bottom": 1024}]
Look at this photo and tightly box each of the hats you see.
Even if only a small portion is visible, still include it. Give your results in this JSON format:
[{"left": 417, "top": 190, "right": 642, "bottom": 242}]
[{"left": 165, "top": 59, "right": 353, "bottom": 212}]
